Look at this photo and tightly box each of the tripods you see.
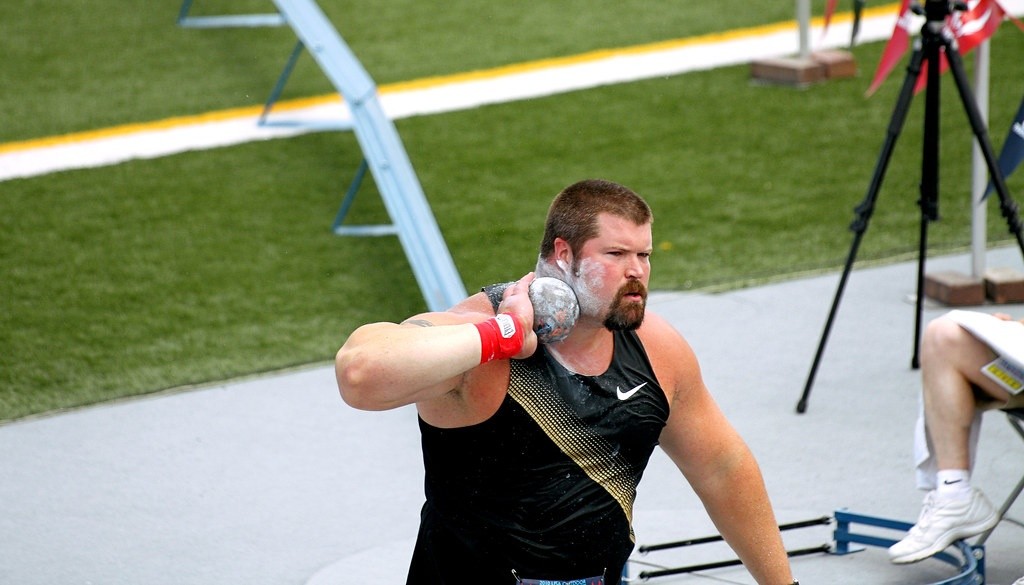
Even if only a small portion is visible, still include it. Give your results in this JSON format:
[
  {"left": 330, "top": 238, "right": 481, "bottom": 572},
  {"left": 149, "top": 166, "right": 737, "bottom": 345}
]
[{"left": 795, "top": 1, "right": 1024, "bottom": 413}]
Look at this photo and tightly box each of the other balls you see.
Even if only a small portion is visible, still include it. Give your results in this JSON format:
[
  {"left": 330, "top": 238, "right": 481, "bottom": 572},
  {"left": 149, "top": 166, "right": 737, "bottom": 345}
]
[{"left": 529, "top": 276, "right": 580, "bottom": 344}]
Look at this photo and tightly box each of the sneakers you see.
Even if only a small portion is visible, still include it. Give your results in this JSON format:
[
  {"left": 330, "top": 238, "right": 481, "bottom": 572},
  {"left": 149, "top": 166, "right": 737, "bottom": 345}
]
[{"left": 889, "top": 485, "right": 999, "bottom": 563}]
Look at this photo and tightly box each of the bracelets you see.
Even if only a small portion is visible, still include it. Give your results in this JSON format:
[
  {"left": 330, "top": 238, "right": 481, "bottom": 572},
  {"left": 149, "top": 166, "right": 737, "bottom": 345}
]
[{"left": 474, "top": 311, "right": 525, "bottom": 366}]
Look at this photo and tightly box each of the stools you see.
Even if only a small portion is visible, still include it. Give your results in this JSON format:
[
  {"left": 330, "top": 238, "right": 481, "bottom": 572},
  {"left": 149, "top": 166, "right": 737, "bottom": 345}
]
[{"left": 974, "top": 409, "right": 1024, "bottom": 547}]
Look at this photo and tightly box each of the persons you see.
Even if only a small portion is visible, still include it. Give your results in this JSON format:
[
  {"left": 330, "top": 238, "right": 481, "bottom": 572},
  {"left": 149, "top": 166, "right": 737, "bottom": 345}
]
[
  {"left": 888, "top": 307, "right": 1024, "bottom": 567},
  {"left": 334, "top": 180, "right": 803, "bottom": 584}
]
